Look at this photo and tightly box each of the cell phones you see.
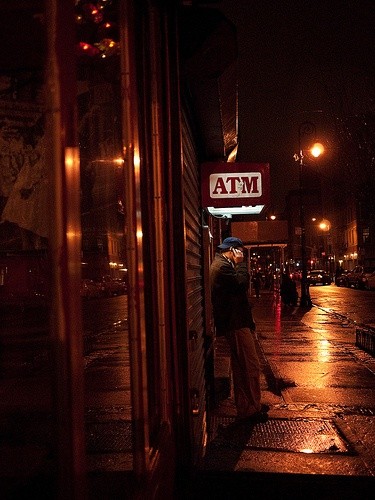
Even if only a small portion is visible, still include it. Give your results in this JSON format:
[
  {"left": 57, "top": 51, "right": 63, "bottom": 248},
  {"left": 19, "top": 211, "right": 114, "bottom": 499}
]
[{"left": 230, "top": 246, "right": 238, "bottom": 253}]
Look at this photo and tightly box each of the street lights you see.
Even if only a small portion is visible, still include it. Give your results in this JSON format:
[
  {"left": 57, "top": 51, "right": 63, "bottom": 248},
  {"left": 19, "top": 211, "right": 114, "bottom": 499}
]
[
  {"left": 320, "top": 221, "right": 333, "bottom": 281},
  {"left": 299, "top": 121, "right": 325, "bottom": 309}
]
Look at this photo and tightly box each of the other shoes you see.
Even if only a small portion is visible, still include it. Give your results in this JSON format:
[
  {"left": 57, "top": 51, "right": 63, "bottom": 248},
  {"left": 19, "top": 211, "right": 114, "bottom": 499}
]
[{"left": 236, "top": 403, "right": 269, "bottom": 424}]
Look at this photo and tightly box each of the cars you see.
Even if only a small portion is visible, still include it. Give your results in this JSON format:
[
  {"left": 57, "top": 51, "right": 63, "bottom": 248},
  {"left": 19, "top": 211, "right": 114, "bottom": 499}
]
[
  {"left": 80, "top": 274, "right": 128, "bottom": 302},
  {"left": 307, "top": 269, "right": 332, "bottom": 285},
  {"left": 336, "top": 272, "right": 375, "bottom": 289}
]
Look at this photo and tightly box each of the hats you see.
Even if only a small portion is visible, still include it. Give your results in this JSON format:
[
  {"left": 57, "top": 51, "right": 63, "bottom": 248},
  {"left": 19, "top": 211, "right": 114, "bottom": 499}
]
[{"left": 217, "top": 237, "right": 242, "bottom": 248}]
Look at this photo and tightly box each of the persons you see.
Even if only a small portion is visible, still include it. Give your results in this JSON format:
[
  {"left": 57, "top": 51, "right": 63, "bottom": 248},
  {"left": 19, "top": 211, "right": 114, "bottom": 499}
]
[
  {"left": 210, "top": 237, "right": 270, "bottom": 418},
  {"left": 334, "top": 265, "right": 344, "bottom": 285},
  {"left": 253, "top": 269, "right": 271, "bottom": 298},
  {"left": 279, "top": 274, "right": 299, "bottom": 304}
]
